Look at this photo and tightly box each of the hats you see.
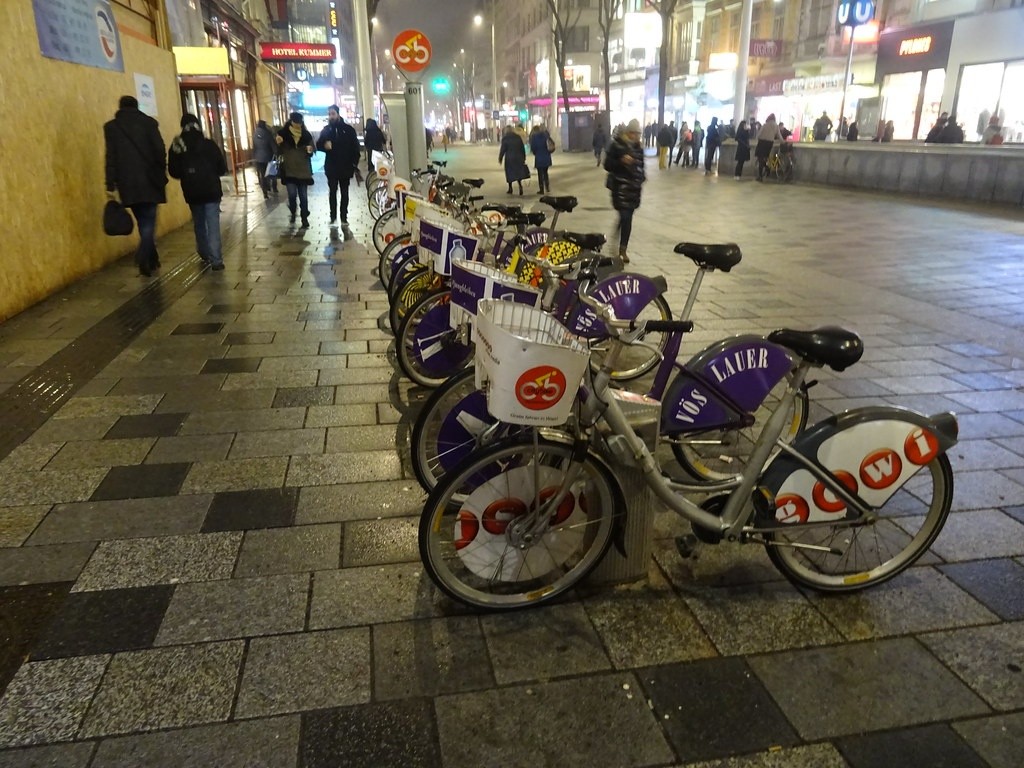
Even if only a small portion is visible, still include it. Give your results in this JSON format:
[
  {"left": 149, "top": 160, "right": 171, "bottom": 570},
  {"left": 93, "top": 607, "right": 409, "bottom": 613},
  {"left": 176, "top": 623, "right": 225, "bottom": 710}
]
[
  {"left": 628, "top": 120, "right": 642, "bottom": 133},
  {"left": 291, "top": 112, "right": 302, "bottom": 123}
]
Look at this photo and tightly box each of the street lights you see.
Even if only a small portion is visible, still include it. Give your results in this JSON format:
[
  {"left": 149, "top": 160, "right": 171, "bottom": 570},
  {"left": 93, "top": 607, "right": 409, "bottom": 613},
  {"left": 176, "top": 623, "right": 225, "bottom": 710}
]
[{"left": 474, "top": 16, "right": 498, "bottom": 144}]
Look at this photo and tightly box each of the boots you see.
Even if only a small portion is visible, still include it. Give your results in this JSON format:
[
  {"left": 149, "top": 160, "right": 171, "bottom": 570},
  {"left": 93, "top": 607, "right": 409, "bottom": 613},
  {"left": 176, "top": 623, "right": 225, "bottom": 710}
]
[
  {"left": 545, "top": 180, "right": 549, "bottom": 191},
  {"left": 537, "top": 185, "right": 544, "bottom": 194}
]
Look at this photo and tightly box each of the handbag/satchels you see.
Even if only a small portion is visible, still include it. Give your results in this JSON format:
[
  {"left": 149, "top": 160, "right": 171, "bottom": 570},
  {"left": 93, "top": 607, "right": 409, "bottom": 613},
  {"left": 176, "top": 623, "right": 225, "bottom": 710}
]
[
  {"left": 546, "top": 138, "right": 555, "bottom": 153},
  {"left": 264, "top": 153, "right": 280, "bottom": 177},
  {"left": 103, "top": 202, "right": 133, "bottom": 236}
]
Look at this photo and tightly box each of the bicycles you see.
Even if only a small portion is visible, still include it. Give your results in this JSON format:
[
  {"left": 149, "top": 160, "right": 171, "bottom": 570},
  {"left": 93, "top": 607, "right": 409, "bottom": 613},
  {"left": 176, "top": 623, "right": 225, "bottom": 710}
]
[
  {"left": 418, "top": 253, "right": 961, "bottom": 614},
  {"left": 754, "top": 143, "right": 792, "bottom": 182},
  {"left": 365, "top": 147, "right": 820, "bottom": 514}
]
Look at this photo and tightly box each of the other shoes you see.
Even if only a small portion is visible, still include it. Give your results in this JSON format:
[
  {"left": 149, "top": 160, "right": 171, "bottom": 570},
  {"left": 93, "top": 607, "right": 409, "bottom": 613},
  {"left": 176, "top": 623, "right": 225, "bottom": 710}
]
[
  {"left": 290, "top": 214, "right": 294, "bottom": 223},
  {"left": 520, "top": 190, "right": 523, "bottom": 195},
  {"left": 507, "top": 187, "right": 512, "bottom": 193},
  {"left": 302, "top": 217, "right": 309, "bottom": 227},
  {"left": 342, "top": 219, "right": 349, "bottom": 226},
  {"left": 212, "top": 263, "right": 226, "bottom": 269},
  {"left": 140, "top": 259, "right": 161, "bottom": 276},
  {"left": 330, "top": 215, "right": 336, "bottom": 223}
]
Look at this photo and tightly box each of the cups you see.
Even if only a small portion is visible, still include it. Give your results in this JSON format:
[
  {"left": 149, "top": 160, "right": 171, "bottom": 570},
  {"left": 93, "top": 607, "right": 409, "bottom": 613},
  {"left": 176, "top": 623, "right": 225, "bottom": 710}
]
[
  {"left": 306, "top": 146, "right": 312, "bottom": 153},
  {"left": 327, "top": 141, "right": 332, "bottom": 149}
]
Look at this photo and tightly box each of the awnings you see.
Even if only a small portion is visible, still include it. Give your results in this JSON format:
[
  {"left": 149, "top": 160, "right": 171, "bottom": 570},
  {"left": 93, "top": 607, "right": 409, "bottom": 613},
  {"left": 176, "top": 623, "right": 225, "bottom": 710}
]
[{"left": 179, "top": 75, "right": 227, "bottom": 89}]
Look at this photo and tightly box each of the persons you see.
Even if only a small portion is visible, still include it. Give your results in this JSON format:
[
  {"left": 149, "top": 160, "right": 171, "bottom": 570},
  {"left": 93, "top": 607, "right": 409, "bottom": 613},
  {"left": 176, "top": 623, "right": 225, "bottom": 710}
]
[
  {"left": 603, "top": 119, "right": 646, "bottom": 263},
  {"left": 755, "top": 113, "right": 792, "bottom": 182},
  {"left": 593, "top": 124, "right": 605, "bottom": 167},
  {"left": 103, "top": 95, "right": 169, "bottom": 277},
  {"left": 925, "top": 112, "right": 963, "bottom": 142},
  {"left": 316, "top": 104, "right": 361, "bottom": 227},
  {"left": 498, "top": 123, "right": 555, "bottom": 195},
  {"left": 168, "top": 114, "right": 226, "bottom": 271},
  {"left": 364, "top": 118, "right": 390, "bottom": 174},
  {"left": 734, "top": 121, "right": 750, "bottom": 181},
  {"left": 982, "top": 116, "right": 1001, "bottom": 144},
  {"left": 276, "top": 112, "right": 316, "bottom": 227},
  {"left": 875, "top": 120, "right": 894, "bottom": 142},
  {"left": 253, "top": 120, "right": 283, "bottom": 199},
  {"left": 426, "top": 126, "right": 456, "bottom": 158},
  {"left": 813, "top": 111, "right": 859, "bottom": 142},
  {"left": 704, "top": 116, "right": 761, "bottom": 176},
  {"left": 641, "top": 120, "right": 704, "bottom": 170},
  {"left": 471, "top": 126, "right": 500, "bottom": 143}
]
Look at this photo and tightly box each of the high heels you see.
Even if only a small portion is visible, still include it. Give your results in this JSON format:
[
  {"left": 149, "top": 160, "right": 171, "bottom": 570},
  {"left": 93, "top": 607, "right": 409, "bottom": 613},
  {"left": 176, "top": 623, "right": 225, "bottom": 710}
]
[{"left": 619, "top": 248, "right": 630, "bottom": 263}]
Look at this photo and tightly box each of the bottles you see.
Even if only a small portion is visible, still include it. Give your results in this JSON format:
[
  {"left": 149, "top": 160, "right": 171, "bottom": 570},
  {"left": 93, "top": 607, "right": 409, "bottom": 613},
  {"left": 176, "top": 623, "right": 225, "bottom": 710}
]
[{"left": 449, "top": 240, "right": 466, "bottom": 262}]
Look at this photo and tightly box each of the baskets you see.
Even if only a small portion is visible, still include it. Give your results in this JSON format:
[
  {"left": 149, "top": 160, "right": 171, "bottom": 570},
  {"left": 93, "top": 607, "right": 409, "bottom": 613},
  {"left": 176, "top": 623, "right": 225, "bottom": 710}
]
[
  {"left": 370, "top": 150, "right": 451, "bottom": 230},
  {"left": 412, "top": 207, "right": 464, "bottom": 248},
  {"left": 475, "top": 297, "right": 592, "bottom": 427},
  {"left": 419, "top": 220, "right": 480, "bottom": 276},
  {"left": 449, "top": 260, "right": 542, "bottom": 343}
]
